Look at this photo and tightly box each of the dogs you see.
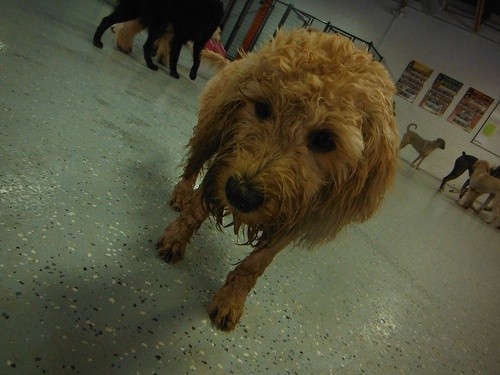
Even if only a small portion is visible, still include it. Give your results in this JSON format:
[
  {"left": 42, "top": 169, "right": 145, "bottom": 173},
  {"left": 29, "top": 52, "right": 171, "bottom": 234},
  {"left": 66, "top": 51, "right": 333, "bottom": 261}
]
[
  {"left": 156, "top": 25, "right": 401, "bottom": 333},
  {"left": 439, "top": 151, "right": 500, "bottom": 213},
  {"left": 458, "top": 160, "right": 500, "bottom": 228},
  {"left": 92, "top": 0, "right": 224, "bottom": 81},
  {"left": 398, "top": 123, "right": 446, "bottom": 171}
]
